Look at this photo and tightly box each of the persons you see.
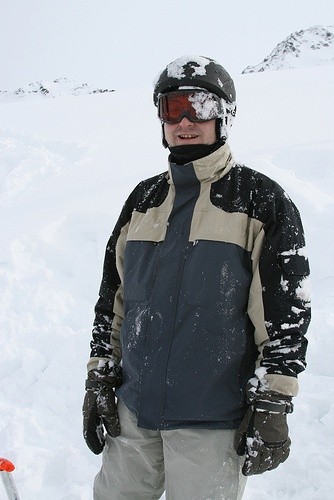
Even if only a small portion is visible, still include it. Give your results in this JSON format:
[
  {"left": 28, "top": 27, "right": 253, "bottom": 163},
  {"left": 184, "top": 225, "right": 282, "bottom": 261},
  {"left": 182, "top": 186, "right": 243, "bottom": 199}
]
[{"left": 82, "top": 55, "right": 311, "bottom": 500}]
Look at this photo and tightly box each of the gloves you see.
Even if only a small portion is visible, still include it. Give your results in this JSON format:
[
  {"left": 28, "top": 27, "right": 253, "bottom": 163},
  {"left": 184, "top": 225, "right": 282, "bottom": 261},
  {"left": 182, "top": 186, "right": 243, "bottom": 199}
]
[
  {"left": 233, "top": 388, "right": 294, "bottom": 476},
  {"left": 83, "top": 362, "right": 123, "bottom": 455}
]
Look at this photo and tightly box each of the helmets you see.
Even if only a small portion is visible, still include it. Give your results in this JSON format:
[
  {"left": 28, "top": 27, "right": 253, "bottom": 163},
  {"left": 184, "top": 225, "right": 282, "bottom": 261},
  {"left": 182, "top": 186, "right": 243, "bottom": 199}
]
[{"left": 153, "top": 56, "right": 236, "bottom": 147}]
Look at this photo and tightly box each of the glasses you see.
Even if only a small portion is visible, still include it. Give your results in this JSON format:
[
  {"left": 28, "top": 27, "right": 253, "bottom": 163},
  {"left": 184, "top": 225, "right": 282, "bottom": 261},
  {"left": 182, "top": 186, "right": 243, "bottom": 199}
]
[{"left": 158, "top": 90, "right": 221, "bottom": 124}]
